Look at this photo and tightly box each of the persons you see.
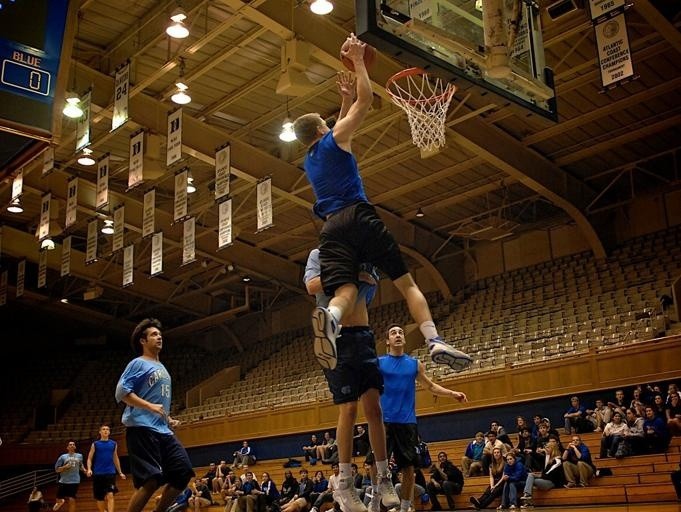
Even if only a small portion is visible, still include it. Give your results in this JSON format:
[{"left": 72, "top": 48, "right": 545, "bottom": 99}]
[
  {"left": 586, "top": 383, "right": 680, "bottom": 459},
  {"left": 426, "top": 451, "right": 464, "bottom": 509},
  {"left": 26, "top": 486, "right": 46, "bottom": 511},
  {"left": 186, "top": 461, "right": 279, "bottom": 509},
  {"left": 268, "top": 459, "right": 376, "bottom": 509},
  {"left": 353, "top": 425, "right": 370, "bottom": 457},
  {"left": 231, "top": 441, "right": 257, "bottom": 469},
  {"left": 373, "top": 327, "right": 470, "bottom": 511},
  {"left": 290, "top": 31, "right": 474, "bottom": 374},
  {"left": 561, "top": 434, "right": 595, "bottom": 488},
  {"left": 301, "top": 222, "right": 406, "bottom": 512},
  {"left": 388, "top": 435, "right": 432, "bottom": 509},
  {"left": 564, "top": 396, "right": 588, "bottom": 435},
  {"left": 460, "top": 414, "right": 563, "bottom": 509},
  {"left": 86, "top": 424, "right": 127, "bottom": 512},
  {"left": 301, "top": 432, "right": 338, "bottom": 465},
  {"left": 116, "top": 318, "right": 194, "bottom": 512},
  {"left": 50, "top": 440, "right": 86, "bottom": 511}
]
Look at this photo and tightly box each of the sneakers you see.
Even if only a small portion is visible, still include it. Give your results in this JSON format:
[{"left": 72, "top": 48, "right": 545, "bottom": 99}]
[
  {"left": 431, "top": 505, "right": 455, "bottom": 511},
  {"left": 332, "top": 476, "right": 368, "bottom": 512},
  {"left": 509, "top": 504, "right": 518, "bottom": 509},
  {"left": 309, "top": 506, "right": 319, "bottom": 512},
  {"left": 426, "top": 336, "right": 474, "bottom": 371},
  {"left": 312, "top": 306, "right": 343, "bottom": 370},
  {"left": 376, "top": 471, "right": 401, "bottom": 508},
  {"left": 563, "top": 483, "right": 576, "bottom": 488},
  {"left": 576, "top": 482, "right": 591, "bottom": 487},
  {"left": 467, "top": 496, "right": 483, "bottom": 511},
  {"left": 230, "top": 464, "right": 243, "bottom": 469},
  {"left": 520, "top": 503, "right": 534, "bottom": 508},
  {"left": 496, "top": 504, "right": 506, "bottom": 509},
  {"left": 53, "top": 499, "right": 65, "bottom": 511},
  {"left": 388, "top": 507, "right": 399, "bottom": 512},
  {"left": 520, "top": 493, "right": 532, "bottom": 500}
]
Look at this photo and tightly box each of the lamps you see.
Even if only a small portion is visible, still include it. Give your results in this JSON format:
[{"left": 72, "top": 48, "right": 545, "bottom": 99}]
[
  {"left": 6, "top": 171, "right": 24, "bottom": 214},
  {"left": 61, "top": 6, "right": 83, "bottom": 119},
  {"left": 305, "top": 0, "right": 334, "bottom": 15},
  {"left": 276, "top": 92, "right": 298, "bottom": 143},
  {"left": 166, "top": 5, "right": 190, "bottom": 40},
  {"left": 187, "top": 165, "right": 197, "bottom": 194},
  {"left": 170, "top": 58, "right": 191, "bottom": 106},
  {"left": 101, "top": 166, "right": 115, "bottom": 234},
  {"left": 77, "top": 83, "right": 98, "bottom": 167}
]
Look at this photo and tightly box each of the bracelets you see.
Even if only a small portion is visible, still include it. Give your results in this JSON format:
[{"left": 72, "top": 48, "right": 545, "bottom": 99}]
[
  {"left": 565, "top": 449, "right": 570, "bottom": 451},
  {"left": 119, "top": 471, "right": 123, "bottom": 475}
]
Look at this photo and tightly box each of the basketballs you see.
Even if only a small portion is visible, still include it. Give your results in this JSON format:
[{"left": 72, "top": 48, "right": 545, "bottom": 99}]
[{"left": 341, "top": 38, "right": 375, "bottom": 71}]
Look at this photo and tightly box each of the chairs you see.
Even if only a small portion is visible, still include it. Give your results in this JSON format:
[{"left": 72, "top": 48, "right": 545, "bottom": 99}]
[{"left": 0, "top": 228, "right": 681, "bottom": 445}]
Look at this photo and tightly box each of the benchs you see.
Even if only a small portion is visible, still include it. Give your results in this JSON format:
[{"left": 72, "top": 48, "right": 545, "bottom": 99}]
[{"left": 0, "top": 426, "right": 681, "bottom": 512}]
